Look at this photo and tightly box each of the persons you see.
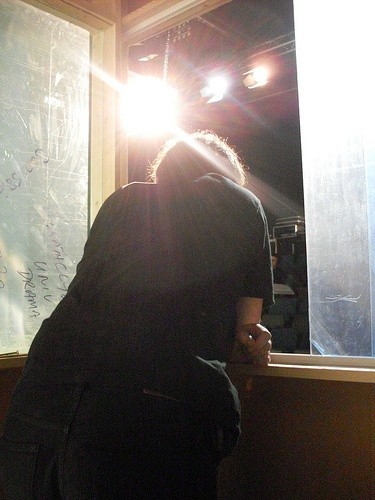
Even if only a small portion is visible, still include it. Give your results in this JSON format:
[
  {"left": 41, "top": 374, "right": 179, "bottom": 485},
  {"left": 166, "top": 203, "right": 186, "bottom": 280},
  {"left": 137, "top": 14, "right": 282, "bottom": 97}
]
[
  {"left": 271, "top": 253, "right": 286, "bottom": 285},
  {"left": 0, "top": 129, "right": 276, "bottom": 500}
]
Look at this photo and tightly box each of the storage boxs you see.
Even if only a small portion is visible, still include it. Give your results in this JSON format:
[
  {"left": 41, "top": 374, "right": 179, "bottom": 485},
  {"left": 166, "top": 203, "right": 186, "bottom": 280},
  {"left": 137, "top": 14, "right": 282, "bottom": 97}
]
[{"left": 270, "top": 216, "right": 306, "bottom": 255}]
